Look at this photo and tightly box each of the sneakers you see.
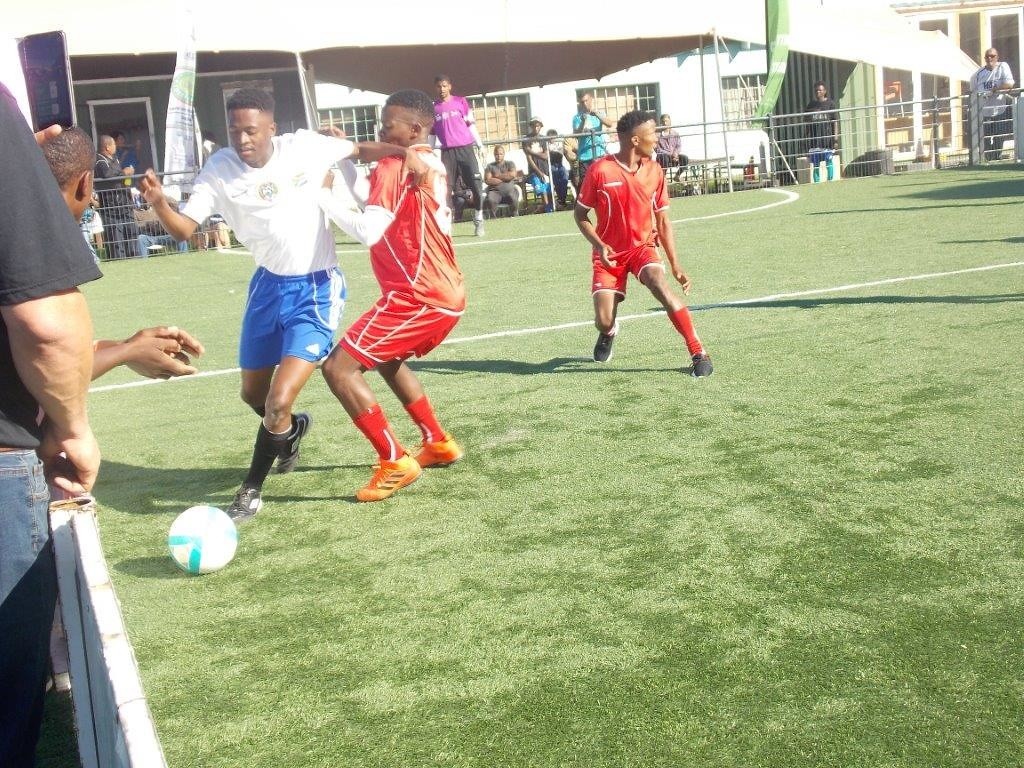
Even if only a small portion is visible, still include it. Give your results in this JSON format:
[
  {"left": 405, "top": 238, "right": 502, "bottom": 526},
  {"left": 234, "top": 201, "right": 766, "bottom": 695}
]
[
  {"left": 414, "top": 439, "right": 464, "bottom": 467},
  {"left": 226, "top": 481, "right": 263, "bottom": 519},
  {"left": 594, "top": 333, "right": 615, "bottom": 363},
  {"left": 690, "top": 354, "right": 714, "bottom": 378},
  {"left": 277, "top": 412, "right": 313, "bottom": 472},
  {"left": 356, "top": 450, "right": 424, "bottom": 501}
]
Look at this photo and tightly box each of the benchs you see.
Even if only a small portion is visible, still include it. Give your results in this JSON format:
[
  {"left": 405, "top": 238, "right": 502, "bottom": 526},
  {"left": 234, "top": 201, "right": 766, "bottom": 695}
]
[{"left": 669, "top": 156, "right": 735, "bottom": 193}]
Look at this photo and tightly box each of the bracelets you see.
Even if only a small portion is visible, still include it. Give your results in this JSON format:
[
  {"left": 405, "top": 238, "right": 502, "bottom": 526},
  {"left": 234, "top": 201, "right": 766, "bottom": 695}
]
[{"left": 833, "top": 140, "right": 838, "bottom": 144}]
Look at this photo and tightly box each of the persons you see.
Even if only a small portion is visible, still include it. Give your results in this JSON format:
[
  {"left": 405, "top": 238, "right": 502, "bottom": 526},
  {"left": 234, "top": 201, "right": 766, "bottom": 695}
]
[
  {"left": 77, "top": 74, "right": 692, "bottom": 258},
  {"left": 141, "top": 84, "right": 430, "bottom": 523},
  {"left": 33, "top": 126, "right": 202, "bottom": 382},
  {"left": 801, "top": 80, "right": 840, "bottom": 182},
  {"left": 0, "top": 85, "right": 101, "bottom": 768},
  {"left": 970, "top": 46, "right": 1015, "bottom": 161},
  {"left": 317, "top": 89, "right": 465, "bottom": 502},
  {"left": 574, "top": 110, "right": 714, "bottom": 378}
]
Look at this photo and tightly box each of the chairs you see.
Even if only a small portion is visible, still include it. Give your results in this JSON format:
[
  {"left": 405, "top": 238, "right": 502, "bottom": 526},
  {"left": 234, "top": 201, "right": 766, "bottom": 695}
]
[
  {"left": 498, "top": 170, "right": 528, "bottom": 216},
  {"left": 885, "top": 81, "right": 905, "bottom": 118}
]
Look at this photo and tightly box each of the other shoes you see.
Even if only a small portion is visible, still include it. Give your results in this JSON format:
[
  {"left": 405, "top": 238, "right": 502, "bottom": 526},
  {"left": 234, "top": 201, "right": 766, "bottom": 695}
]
[
  {"left": 674, "top": 175, "right": 688, "bottom": 186},
  {"left": 544, "top": 202, "right": 553, "bottom": 212},
  {"left": 535, "top": 206, "right": 544, "bottom": 214},
  {"left": 556, "top": 202, "right": 564, "bottom": 210},
  {"left": 568, "top": 204, "right": 575, "bottom": 210},
  {"left": 474, "top": 220, "right": 484, "bottom": 236}
]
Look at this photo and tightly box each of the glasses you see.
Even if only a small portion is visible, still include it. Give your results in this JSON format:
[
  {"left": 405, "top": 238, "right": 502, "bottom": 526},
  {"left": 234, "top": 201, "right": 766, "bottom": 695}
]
[{"left": 985, "top": 55, "right": 997, "bottom": 59}]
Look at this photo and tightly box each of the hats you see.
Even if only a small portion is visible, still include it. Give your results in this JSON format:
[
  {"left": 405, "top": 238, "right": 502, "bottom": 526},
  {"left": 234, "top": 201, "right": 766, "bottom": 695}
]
[{"left": 530, "top": 117, "right": 544, "bottom": 127}]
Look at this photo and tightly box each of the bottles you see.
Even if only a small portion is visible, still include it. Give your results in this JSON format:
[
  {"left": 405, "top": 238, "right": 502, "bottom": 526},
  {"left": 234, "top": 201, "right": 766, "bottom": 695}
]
[
  {"left": 748, "top": 156, "right": 754, "bottom": 179},
  {"left": 124, "top": 165, "right": 133, "bottom": 185}
]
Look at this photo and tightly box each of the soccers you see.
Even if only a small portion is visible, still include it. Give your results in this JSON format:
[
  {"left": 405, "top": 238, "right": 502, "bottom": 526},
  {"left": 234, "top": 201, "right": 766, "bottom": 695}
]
[{"left": 166, "top": 504, "right": 239, "bottom": 575}]
